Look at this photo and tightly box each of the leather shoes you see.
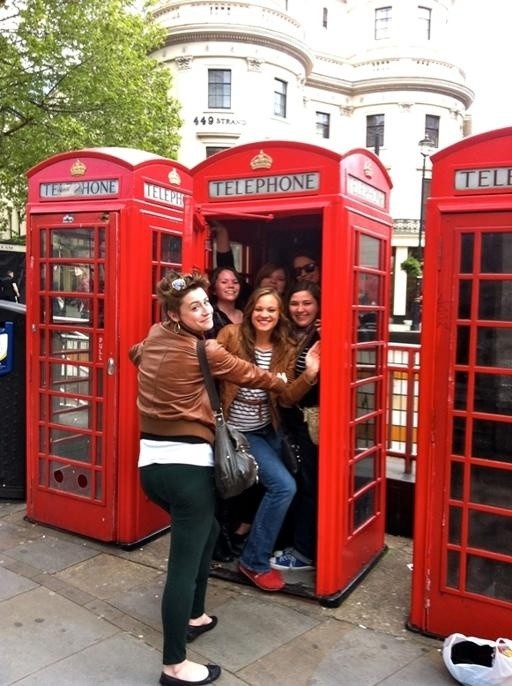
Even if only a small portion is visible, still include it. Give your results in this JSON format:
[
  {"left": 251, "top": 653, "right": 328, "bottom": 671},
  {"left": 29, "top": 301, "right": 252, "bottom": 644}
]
[{"left": 214, "top": 525, "right": 249, "bottom": 562}]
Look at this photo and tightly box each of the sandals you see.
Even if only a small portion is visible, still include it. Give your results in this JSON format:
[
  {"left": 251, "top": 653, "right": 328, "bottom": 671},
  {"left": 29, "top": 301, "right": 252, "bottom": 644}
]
[
  {"left": 160, "top": 665, "right": 221, "bottom": 686},
  {"left": 187, "top": 616, "right": 218, "bottom": 642}
]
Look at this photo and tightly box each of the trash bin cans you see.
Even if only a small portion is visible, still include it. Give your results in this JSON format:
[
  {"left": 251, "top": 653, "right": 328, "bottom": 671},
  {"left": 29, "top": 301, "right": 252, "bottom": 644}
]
[{"left": 1, "top": 301, "right": 59, "bottom": 504}]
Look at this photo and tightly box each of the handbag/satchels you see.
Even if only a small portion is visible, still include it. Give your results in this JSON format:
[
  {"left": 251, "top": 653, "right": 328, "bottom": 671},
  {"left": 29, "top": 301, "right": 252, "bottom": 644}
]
[
  {"left": 214, "top": 418, "right": 259, "bottom": 499},
  {"left": 304, "top": 407, "right": 320, "bottom": 444},
  {"left": 281, "top": 435, "right": 303, "bottom": 473}
]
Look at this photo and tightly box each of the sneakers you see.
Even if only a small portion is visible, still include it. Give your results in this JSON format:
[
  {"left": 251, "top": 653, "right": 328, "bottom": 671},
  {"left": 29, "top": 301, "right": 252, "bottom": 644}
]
[
  {"left": 239, "top": 559, "right": 285, "bottom": 591},
  {"left": 269, "top": 550, "right": 314, "bottom": 570}
]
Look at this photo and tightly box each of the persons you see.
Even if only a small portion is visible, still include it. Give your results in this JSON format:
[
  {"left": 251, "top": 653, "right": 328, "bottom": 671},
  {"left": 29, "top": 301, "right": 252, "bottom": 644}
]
[
  {"left": 127, "top": 264, "right": 289, "bottom": 686},
  {"left": 79, "top": 263, "right": 106, "bottom": 328},
  {"left": 0, "top": 269, "right": 21, "bottom": 303}
]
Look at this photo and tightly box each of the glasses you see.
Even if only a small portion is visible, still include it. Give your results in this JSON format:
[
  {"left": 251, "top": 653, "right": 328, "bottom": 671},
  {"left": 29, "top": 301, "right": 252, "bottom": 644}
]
[
  {"left": 171, "top": 273, "right": 193, "bottom": 292},
  {"left": 293, "top": 262, "right": 319, "bottom": 276}
]
